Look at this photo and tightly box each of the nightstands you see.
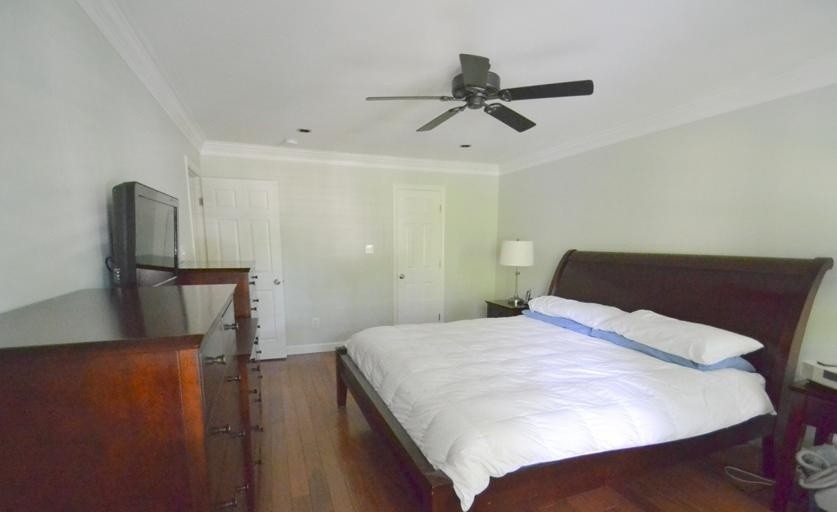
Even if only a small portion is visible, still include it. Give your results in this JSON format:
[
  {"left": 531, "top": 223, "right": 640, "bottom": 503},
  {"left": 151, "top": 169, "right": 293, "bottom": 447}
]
[
  {"left": 775, "top": 377, "right": 836, "bottom": 511},
  {"left": 486, "top": 296, "right": 529, "bottom": 319}
]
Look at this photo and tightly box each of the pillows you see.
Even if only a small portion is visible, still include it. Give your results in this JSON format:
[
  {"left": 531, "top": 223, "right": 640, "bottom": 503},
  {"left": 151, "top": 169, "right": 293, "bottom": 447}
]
[
  {"left": 528, "top": 292, "right": 628, "bottom": 331},
  {"left": 597, "top": 307, "right": 766, "bottom": 371}
]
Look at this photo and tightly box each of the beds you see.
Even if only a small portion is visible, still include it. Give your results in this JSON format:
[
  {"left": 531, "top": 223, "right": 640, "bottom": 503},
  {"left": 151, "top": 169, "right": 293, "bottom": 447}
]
[{"left": 332, "top": 245, "right": 833, "bottom": 511}]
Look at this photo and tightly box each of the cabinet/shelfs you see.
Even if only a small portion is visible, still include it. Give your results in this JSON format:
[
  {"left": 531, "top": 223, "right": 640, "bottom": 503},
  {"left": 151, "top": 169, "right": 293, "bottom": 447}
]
[
  {"left": 178, "top": 260, "right": 264, "bottom": 512},
  {"left": 234, "top": 316, "right": 260, "bottom": 512},
  {"left": 0, "top": 285, "right": 252, "bottom": 512}
]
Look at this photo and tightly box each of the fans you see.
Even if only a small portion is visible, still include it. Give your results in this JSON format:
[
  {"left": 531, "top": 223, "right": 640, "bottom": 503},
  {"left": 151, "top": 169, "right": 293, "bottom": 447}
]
[{"left": 359, "top": 49, "right": 598, "bottom": 137}]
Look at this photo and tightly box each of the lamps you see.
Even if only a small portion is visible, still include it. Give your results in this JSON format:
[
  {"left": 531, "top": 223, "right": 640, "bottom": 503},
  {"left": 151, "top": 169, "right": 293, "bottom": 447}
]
[{"left": 495, "top": 235, "right": 538, "bottom": 308}]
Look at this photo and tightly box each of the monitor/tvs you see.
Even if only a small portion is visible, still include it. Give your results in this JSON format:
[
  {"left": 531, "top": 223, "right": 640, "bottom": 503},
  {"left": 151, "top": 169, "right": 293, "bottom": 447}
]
[{"left": 112, "top": 181, "right": 178, "bottom": 287}]
